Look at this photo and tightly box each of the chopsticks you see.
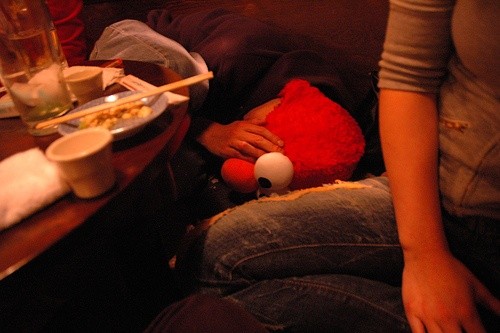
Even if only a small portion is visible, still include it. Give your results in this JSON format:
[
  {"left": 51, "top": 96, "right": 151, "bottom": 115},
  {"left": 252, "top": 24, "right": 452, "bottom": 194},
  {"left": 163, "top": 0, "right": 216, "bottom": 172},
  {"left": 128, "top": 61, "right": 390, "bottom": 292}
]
[{"left": 34, "top": 70, "right": 213, "bottom": 129}]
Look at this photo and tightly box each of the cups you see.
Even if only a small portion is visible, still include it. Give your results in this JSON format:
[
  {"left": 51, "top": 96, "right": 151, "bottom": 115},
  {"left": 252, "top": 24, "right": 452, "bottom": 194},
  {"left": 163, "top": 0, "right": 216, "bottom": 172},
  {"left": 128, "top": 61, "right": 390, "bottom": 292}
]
[
  {"left": 46, "top": 127, "right": 117, "bottom": 198},
  {"left": 1, "top": 0, "right": 77, "bottom": 136},
  {"left": 0, "top": 0, "right": 76, "bottom": 103},
  {"left": 64, "top": 70, "right": 103, "bottom": 104}
]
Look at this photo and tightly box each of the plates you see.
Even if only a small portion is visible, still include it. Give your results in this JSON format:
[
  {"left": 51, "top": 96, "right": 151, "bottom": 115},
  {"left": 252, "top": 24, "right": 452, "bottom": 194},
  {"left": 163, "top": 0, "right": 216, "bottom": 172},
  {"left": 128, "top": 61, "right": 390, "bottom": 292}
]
[{"left": 57, "top": 91, "right": 169, "bottom": 141}]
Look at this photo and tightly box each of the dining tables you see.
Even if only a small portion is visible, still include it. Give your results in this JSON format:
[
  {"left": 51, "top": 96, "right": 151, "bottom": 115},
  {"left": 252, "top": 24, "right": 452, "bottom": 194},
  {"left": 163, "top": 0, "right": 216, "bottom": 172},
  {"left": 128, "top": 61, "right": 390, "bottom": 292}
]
[{"left": 0, "top": 59, "right": 190, "bottom": 284}]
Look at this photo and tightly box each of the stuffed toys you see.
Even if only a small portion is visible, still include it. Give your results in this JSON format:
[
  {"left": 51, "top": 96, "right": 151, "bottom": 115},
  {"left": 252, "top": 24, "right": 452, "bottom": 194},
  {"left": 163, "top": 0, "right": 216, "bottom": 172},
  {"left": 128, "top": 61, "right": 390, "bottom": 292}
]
[{"left": 221, "top": 74, "right": 365, "bottom": 195}]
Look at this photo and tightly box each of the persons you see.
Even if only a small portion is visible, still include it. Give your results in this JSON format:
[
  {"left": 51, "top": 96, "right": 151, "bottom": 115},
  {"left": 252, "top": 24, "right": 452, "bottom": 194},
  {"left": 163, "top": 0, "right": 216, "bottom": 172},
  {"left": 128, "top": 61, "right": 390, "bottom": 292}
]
[
  {"left": 178, "top": 0, "right": 500, "bottom": 332},
  {"left": 86, "top": 3, "right": 386, "bottom": 262},
  {"left": 1, "top": 0, "right": 88, "bottom": 86}
]
